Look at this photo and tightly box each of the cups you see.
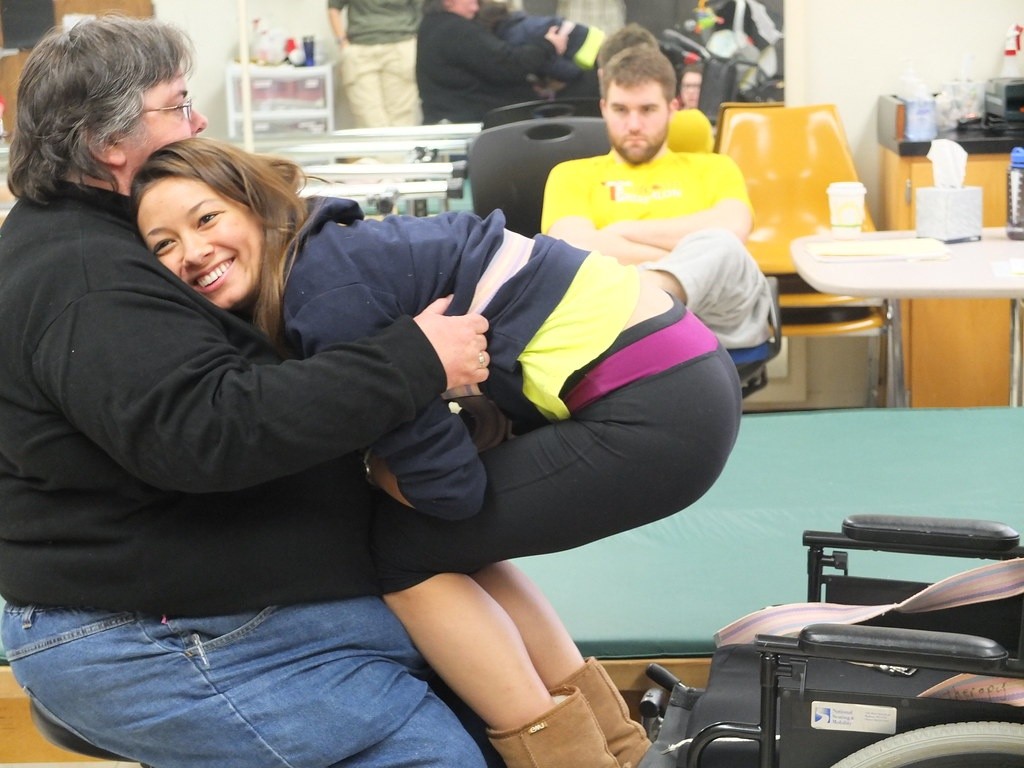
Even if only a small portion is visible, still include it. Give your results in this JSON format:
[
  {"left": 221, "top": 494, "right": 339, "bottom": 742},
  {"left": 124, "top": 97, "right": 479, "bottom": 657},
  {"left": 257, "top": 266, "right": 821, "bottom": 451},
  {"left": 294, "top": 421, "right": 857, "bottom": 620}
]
[
  {"left": 1005, "top": 148, "right": 1024, "bottom": 241},
  {"left": 827, "top": 182, "right": 866, "bottom": 239}
]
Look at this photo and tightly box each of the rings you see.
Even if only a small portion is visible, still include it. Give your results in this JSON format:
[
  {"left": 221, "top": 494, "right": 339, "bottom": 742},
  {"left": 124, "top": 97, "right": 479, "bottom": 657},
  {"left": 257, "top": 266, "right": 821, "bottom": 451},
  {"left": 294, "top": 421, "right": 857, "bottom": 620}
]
[{"left": 477, "top": 353, "right": 485, "bottom": 367}]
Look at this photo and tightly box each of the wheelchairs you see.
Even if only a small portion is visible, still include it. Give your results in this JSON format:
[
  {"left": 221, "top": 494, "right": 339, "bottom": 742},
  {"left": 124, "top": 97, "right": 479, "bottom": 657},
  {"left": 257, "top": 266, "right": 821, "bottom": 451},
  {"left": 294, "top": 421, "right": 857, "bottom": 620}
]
[
  {"left": 642, "top": 513, "right": 1024, "bottom": 768},
  {"left": 664, "top": 26, "right": 785, "bottom": 119}
]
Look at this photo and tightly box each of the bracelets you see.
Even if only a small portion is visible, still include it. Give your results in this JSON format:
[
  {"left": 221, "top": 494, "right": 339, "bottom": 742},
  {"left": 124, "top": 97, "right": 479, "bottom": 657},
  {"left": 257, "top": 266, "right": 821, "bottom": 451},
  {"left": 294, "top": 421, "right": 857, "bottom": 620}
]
[{"left": 336, "top": 35, "right": 347, "bottom": 44}]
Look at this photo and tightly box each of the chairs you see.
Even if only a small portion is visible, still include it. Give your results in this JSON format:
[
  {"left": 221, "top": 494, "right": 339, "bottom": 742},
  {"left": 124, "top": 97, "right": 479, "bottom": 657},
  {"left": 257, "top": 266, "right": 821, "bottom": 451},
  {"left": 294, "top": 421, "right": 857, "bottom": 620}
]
[{"left": 468, "top": 96, "right": 905, "bottom": 410}]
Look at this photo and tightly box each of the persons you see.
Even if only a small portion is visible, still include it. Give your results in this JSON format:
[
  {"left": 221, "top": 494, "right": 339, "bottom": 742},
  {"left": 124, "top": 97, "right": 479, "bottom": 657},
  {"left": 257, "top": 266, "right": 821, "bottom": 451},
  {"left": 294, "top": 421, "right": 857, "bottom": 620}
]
[
  {"left": 540, "top": 42, "right": 774, "bottom": 348},
  {"left": 130, "top": 138, "right": 744, "bottom": 768},
  {"left": 327, "top": 0, "right": 711, "bottom": 128},
  {"left": 0, "top": 13, "right": 509, "bottom": 768}
]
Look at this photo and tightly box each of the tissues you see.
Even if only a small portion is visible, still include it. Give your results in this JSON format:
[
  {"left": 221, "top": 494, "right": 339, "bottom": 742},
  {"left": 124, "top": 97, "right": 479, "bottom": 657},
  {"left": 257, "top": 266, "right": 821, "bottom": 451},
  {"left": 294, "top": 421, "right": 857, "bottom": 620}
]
[{"left": 918, "top": 138, "right": 986, "bottom": 239}]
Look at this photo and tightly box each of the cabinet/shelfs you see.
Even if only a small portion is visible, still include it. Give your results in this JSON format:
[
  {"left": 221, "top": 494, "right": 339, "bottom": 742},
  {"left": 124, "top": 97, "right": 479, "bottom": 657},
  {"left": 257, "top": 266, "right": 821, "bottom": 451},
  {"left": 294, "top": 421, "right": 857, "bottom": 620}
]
[{"left": 225, "top": 41, "right": 346, "bottom": 143}]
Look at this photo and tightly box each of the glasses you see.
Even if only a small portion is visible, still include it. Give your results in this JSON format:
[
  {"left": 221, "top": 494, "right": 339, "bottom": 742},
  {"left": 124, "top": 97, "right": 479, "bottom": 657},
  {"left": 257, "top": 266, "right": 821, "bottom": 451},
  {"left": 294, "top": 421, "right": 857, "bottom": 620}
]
[{"left": 143, "top": 98, "right": 192, "bottom": 119}]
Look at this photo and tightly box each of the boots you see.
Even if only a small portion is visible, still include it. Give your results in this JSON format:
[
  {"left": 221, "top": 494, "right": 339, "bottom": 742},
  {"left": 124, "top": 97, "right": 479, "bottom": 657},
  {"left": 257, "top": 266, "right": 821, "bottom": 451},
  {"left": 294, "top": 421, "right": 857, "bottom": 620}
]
[
  {"left": 486, "top": 685, "right": 620, "bottom": 768},
  {"left": 555, "top": 656, "right": 652, "bottom": 768}
]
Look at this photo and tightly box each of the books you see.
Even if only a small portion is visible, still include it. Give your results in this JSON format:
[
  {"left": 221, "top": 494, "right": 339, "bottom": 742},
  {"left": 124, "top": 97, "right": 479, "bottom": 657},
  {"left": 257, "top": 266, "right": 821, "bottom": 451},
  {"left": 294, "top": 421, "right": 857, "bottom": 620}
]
[{"left": 804, "top": 237, "right": 953, "bottom": 264}]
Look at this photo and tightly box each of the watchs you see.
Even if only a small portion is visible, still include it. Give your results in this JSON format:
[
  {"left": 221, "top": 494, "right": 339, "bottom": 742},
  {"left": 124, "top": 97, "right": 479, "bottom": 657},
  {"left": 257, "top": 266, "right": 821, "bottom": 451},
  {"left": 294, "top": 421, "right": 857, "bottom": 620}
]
[{"left": 361, "top": 447, "right": 377, "bottom": 487}]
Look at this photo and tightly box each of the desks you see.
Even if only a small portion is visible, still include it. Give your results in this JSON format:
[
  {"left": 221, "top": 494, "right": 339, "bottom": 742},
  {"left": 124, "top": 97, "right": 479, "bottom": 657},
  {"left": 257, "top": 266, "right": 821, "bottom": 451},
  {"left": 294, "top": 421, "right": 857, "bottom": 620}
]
[
  {"left": 791, "top": 227, "right": 1024, "bottom": 408},
  {"left": 876, "top": 147, "right": 1024, "bottom": 407}
]
